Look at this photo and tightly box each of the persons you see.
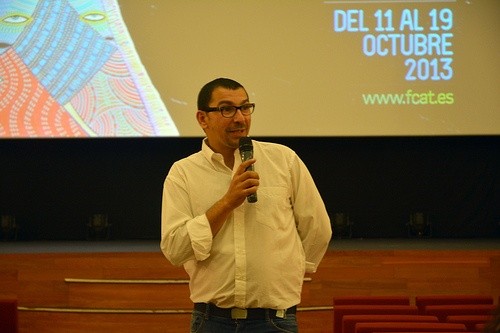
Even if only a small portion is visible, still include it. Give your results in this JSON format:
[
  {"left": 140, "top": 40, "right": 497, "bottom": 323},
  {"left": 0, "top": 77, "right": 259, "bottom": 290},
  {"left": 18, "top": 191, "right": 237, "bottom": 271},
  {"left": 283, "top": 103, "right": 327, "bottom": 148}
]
[{"left": 161, "top": 78, "right": 332, "bottom": 333}]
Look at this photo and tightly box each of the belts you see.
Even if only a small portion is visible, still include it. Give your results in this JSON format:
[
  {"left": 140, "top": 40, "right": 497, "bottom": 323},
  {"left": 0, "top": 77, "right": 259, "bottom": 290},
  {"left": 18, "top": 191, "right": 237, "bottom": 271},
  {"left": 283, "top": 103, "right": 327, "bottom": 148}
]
[{"left": 194, "top": 302, "right": 297, "bottom": 320}]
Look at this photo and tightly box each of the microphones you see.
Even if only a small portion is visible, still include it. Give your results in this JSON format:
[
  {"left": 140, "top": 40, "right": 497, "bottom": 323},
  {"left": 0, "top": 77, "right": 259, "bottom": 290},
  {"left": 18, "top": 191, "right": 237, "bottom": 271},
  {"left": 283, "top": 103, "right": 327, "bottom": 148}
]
[{"left": 239, "top": 136, "right": 257, "bottom": 203}]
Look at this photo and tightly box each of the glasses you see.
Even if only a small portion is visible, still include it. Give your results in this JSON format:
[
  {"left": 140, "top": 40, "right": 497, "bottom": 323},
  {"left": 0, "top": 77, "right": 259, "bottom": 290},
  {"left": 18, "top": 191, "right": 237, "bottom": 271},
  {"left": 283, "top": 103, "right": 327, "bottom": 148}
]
[{"left": 200, "top": 103, "right": 256, "bottom": 118}]
